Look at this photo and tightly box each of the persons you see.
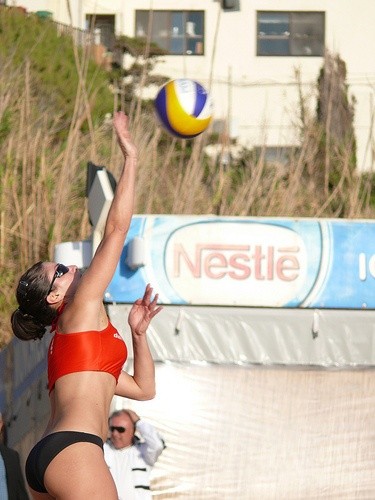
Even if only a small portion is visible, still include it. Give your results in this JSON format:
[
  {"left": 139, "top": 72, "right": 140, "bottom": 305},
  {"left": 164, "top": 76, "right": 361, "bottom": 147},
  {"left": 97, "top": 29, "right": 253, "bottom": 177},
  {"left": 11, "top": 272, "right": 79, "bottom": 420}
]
[
  {"left": 11, "top": 111, "right": 164, "bottom": 500},
  {"left": 103, "top": 409, "right": 167, "bottom": 500}
]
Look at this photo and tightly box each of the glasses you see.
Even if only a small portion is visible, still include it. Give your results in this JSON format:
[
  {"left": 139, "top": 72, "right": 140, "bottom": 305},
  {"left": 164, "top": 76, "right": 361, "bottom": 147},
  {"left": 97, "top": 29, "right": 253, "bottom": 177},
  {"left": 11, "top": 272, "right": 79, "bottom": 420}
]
[
  {"left": 45, "top": 264, "right": 69, "bottom": 300},
  {"left": 109, "top": 426, "right": 131, "bottom": 433}
]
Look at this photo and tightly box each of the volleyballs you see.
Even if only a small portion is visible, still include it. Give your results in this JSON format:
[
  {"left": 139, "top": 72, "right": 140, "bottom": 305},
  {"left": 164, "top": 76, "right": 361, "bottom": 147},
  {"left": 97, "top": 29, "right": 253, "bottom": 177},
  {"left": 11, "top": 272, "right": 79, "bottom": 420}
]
[{"left": 154, "top": 78, "right": 214, "bottom": 139}]
